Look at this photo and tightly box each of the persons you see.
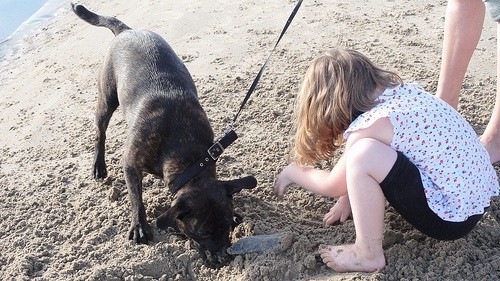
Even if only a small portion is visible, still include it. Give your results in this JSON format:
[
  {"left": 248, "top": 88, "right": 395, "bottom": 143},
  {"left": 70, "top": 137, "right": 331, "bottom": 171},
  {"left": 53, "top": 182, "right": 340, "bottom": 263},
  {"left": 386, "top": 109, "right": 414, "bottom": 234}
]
[
  {"left": 274, "top": 49, "right": 499, "bottom": 273},
  {"left": 434, "top": 0, "right": 500, "bottom": 167}
]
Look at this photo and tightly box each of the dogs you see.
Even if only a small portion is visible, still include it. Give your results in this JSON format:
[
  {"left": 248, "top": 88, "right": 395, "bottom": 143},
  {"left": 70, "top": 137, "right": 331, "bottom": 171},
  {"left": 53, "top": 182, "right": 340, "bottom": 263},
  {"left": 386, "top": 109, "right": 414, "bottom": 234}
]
[{"left": 72, "top": 2, "right": 258, "bottom": 268}]
